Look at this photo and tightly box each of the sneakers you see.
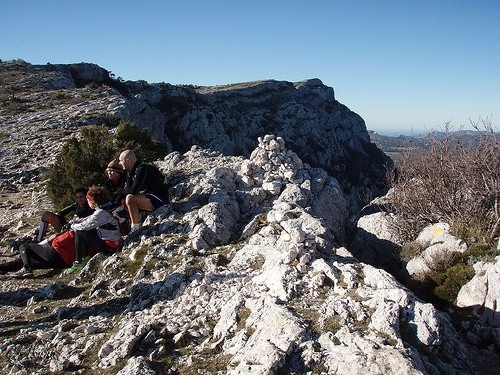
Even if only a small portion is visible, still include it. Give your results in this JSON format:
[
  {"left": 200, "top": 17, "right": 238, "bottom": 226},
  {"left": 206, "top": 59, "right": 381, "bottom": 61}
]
[
  {"left": 64, "top": 262, "right": 80, "bottom": 274},
  {"left": 10, "top": 267, "right": 33, "bottom": 277},
  {"left": 125, "top": 227, "right": 140, "bottom": 241}
]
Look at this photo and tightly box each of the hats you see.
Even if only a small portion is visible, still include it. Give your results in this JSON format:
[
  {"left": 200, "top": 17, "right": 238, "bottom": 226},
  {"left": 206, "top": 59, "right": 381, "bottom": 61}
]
[{"left": 105, "top": 159, "right": 123, "bottom": 173}]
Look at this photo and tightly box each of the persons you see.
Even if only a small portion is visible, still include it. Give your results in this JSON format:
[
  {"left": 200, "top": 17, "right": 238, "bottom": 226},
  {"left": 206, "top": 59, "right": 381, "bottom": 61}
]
[
  {"left": 111, "top": 149, "right": 167, "bottom": 235},
  {"left": 0, "top": 228, "right": 97, "bottom": 278},
  {"left": 30, "top": 188, "right": 95, "bottom": 243},
  {"left": 100, "top": 159, "right": 132, "bottom": 212},
  {"left": 66, "top": 187, "right": 122, "bottom": 270}
]
